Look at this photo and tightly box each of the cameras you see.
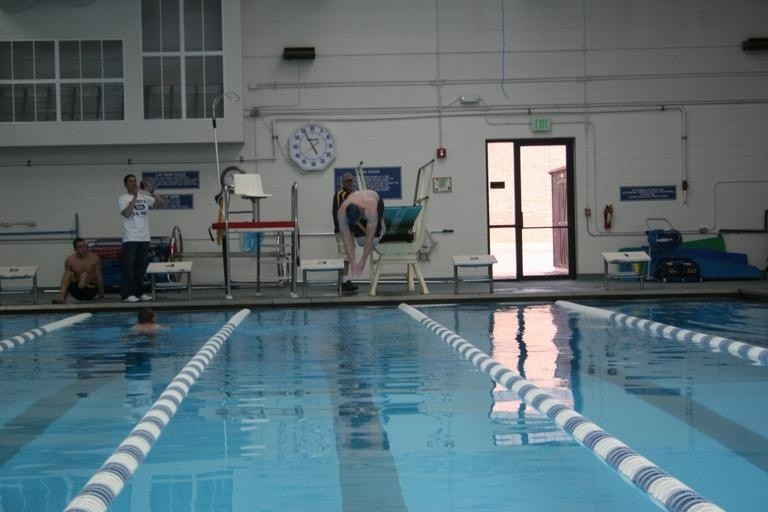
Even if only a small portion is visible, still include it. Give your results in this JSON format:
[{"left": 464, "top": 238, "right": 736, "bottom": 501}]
[{"left": 140, "top": 181, "right": 144, "bottom": 189}]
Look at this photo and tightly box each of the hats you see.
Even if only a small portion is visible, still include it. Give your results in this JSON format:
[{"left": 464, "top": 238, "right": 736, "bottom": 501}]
[
  {"left": 342, "top": 172, "right": 353, "bottom": 181},
  {"left": 345, "top": 204, "right": 359, "bottom": 220}
]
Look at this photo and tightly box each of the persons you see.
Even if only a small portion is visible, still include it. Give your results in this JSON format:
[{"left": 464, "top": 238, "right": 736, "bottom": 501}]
[
  {"left": 337, "top": 189, "right": 385, "bottom": 278},
  {"left": 126, "top": 307, "right": 168, "bottom": 334},
  {"left": 53, "top": 318, "right": 106, "bottom": 378},
  {"left": 117, "top": 335, "right": 156, "bottom": 432},
  {"left": 50, "top": 239, "right": 106, "bottom": 304},
  {"left": 117, "top": 171, "right": 166, "bottom": 304},
  {"left": 332, "top": 172, "right": 360, "bottom": 291}
]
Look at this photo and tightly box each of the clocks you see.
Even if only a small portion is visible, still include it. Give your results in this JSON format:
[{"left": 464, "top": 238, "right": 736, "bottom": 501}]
[
  {"left": 220, "top": 166, "right": 244, "bottom": 194},
  {"left": 285, "top": 122, "right": 340, "bottom": 176}
]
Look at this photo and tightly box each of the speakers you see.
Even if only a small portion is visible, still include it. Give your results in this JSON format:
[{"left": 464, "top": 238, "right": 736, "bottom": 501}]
[
  {"left": 284, "top": 46, "right": 316, "bottom": 61},
  {"left": 741, "top": 38, "right": 768, "bottom": 51}
]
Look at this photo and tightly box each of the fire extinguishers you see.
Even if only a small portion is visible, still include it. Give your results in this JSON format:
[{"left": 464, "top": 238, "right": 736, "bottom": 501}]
[{"left": 603, "top": 204, "right": 613, "bottom": 229}]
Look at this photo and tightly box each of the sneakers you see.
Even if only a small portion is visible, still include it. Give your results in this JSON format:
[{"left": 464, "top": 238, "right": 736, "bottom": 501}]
[
  {"left": 338, "top": 280, "right": 358, "bottom": 291},
  {"left": 140, "top": 294, "right": 152, "bottom": 301},
  {"left": 121, "top": 295, "right": 140, "bottom": 302}
]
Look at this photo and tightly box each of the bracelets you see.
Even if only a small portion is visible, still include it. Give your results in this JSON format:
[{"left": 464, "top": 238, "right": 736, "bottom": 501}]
[
  {"left": 148, "top": 187, "right": 155, "bottom": 195},
  {"left": 97, "top": 295, "right": 104, "bottom": 300}
]
